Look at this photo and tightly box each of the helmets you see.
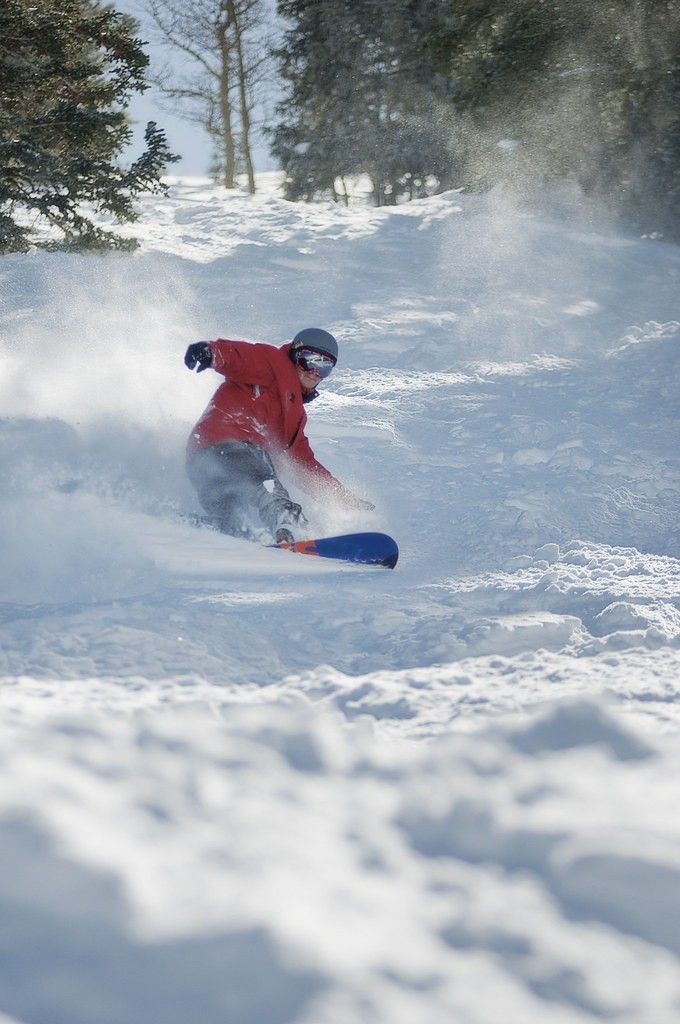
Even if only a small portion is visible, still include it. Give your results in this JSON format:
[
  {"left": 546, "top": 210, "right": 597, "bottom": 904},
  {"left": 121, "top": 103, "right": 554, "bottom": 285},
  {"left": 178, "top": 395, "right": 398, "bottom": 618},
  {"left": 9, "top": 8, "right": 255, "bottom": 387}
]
[{"left": 289, "top": 328, "right": 338, "bottom": 367}]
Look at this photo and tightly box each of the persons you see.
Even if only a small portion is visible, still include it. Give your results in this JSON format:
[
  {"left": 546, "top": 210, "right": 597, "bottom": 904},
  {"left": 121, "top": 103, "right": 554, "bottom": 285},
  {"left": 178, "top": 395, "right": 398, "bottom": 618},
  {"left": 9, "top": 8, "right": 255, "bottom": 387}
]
[{"left": 184, "top": 327, "right": 375, "bottom": 545}]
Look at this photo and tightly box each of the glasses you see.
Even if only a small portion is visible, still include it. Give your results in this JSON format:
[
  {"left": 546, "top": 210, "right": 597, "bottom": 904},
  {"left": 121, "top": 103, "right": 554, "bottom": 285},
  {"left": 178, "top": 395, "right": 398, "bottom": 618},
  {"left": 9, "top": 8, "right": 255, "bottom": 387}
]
[{"left": 295, "top": 350, "right": 335, "bottom": 378}]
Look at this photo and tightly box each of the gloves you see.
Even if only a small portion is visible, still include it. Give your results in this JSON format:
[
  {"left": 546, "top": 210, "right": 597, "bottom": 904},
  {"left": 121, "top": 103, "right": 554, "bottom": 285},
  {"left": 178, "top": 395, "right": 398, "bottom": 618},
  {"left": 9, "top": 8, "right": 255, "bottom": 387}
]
[
  {"left": 184, "top": 342, "right": 213, "bottom": 372},
  {"left": 352, "top": 500, "right": 374, "bottom": 510}
]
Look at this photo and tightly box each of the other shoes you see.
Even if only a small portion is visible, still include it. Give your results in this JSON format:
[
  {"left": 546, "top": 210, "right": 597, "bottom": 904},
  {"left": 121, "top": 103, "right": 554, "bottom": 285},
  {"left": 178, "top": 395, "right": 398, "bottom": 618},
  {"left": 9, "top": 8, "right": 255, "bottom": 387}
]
[{"left": 275, "top": 524, "right": 308, "bottom": 544}]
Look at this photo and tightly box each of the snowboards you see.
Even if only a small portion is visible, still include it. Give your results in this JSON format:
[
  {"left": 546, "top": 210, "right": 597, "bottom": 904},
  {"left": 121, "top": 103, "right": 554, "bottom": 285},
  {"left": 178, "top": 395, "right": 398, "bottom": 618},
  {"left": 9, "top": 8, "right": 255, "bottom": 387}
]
[{"left": 263, "top": 531, "right": 400, "bottom": 572}]
